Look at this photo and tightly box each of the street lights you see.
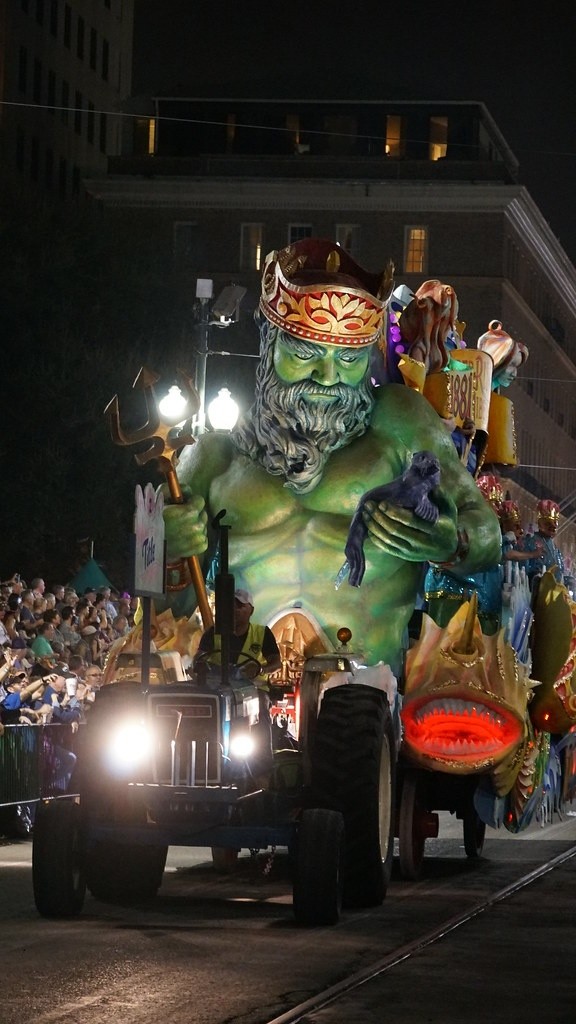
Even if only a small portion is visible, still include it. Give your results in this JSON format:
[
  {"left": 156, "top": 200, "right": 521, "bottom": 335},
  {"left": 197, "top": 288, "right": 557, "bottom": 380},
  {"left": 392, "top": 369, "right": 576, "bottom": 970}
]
[{"left": 160, "top": 276, "right": 248, "bottom": 438}]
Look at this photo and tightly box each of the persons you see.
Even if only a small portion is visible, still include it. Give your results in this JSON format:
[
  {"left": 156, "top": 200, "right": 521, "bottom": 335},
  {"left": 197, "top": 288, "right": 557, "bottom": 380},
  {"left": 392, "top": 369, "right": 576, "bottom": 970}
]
[
  {"left": 193, "top": 588, "right": 283, "bottom": 789},
  {"left": 0, "top": 576, "right": 137, "bottom": 837},
  {"left": 150, "top": 236, "right": 528, "bottom": 682},
  {"left": 425, "top": 476, "right": 576, "bottom": 622}
]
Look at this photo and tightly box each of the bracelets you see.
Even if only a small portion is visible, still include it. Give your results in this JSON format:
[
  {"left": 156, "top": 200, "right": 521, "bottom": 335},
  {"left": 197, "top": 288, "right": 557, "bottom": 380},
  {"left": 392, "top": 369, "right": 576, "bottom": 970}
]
[{"left": 42, "top": 678, "right": 45, "bottom": 682}]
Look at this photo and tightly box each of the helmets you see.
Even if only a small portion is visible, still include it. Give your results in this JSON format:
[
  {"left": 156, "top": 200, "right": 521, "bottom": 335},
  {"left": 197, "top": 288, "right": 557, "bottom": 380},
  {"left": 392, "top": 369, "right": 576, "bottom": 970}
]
[{"left": 81, "top": 625, "right": 97, "bottom": 636}]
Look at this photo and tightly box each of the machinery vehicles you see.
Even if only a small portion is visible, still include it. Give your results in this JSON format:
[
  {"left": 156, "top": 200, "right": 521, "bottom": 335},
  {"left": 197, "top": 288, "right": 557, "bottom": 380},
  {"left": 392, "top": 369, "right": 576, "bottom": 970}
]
[{"left": 30, "top": 506, "right": 489, "bottom": 932}]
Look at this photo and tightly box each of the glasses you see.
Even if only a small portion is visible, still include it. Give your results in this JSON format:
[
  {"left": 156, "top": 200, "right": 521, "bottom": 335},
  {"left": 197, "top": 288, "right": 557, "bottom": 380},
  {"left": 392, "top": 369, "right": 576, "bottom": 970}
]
[
  {"left": 11, "top": 681, "right": 27, "bottom": 687},
  {"left": 87, "top": 673, "right": 103, "bottom": 678}
]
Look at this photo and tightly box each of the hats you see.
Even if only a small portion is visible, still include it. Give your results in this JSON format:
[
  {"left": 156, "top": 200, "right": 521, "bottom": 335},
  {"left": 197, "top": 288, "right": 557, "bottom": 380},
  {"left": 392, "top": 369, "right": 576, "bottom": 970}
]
[
  {"left": 234, "top": 587, "right": 253, "bottom": 608},
  {"left": 119, "top": 592, "right": 132, "bottom": 598},
  {"left": 9, "top": 638, "right": 28, "bottom": 649},
  {"left": 84, "top": 586, "right": 96, "bottom": 594},
  {"left": 476, "top": 474, "right": 521, "bottom": 520},
  {"left": 50, "top": 667, "right": 76, "bottom": 678},
  {"left": 536, "top": 500, "right": 559, "bottom": 522},
  {"left": 3, "top": 673, "right": 25, "bottom": 689}
]
